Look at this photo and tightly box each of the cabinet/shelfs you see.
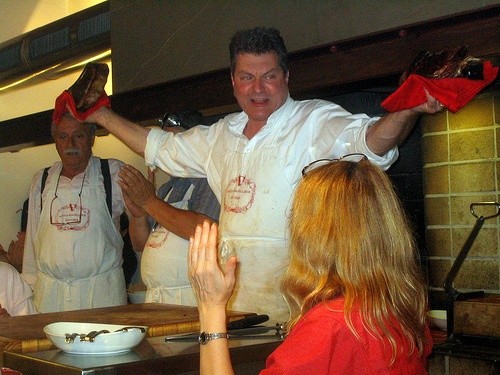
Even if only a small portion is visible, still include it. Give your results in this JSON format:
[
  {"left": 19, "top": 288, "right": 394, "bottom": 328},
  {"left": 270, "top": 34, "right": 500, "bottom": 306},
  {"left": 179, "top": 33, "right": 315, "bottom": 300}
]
[{"left": 0, "top": 303, "right": 284, "bottom": 375}]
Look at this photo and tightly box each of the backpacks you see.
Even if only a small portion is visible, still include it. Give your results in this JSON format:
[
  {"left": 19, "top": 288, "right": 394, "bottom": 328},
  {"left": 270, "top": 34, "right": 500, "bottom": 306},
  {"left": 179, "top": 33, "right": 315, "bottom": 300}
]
[{"left": 41, "top": 159, "right": 137, "bottom": 289}]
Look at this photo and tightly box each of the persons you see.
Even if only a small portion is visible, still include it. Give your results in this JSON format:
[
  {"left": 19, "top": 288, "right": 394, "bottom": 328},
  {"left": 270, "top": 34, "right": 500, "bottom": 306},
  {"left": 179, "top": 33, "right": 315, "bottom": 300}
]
[
  {"left": 188, "top": 152, "right": 434, "bottom": 375},
  {"left": 65, "top": 26, "right": 446, "bottom": 332},
  {"left": 0, "top": 112, "right": 132, "bottom": 319},
  {"left": 117, "top": 108, "right": 220, "bottom": 308}
]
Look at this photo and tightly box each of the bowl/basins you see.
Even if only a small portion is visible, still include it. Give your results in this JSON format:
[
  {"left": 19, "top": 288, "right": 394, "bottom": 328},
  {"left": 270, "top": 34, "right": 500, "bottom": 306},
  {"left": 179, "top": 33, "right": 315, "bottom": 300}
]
[
  {"left": 43, "top": 321, "right": 148, "bottom": 355},
  {"left": 426, "top": 310, "right": 447, "bottom": 331}
]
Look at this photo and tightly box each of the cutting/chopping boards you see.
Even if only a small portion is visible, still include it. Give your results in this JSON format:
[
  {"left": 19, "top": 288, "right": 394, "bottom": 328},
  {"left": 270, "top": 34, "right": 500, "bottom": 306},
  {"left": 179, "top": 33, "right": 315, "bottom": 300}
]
[{"left": 1, "top": 302, "right": 258, "bottom": 352}]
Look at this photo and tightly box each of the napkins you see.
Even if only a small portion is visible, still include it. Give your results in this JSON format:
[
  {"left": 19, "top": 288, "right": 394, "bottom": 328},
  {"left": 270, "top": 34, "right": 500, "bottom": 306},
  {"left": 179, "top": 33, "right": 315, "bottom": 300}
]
[
  {"left": 52, "top": 91, "right": 111, "bottom": 124},
  {"left": 382, "top": 61, "right": 500, "bottom": 114}
]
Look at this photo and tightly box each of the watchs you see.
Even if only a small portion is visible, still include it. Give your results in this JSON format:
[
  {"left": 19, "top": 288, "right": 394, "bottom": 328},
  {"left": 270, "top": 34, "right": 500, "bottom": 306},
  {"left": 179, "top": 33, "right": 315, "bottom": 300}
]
[{"left": 198, "top": 331, "right": 230, "bottom": 345}]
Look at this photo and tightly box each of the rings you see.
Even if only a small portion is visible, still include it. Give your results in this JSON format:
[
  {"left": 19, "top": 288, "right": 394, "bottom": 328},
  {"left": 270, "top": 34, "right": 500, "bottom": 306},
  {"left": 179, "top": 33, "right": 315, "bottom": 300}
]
[{"left": 439, "top": 103, "right": 446, "bottom": 109}]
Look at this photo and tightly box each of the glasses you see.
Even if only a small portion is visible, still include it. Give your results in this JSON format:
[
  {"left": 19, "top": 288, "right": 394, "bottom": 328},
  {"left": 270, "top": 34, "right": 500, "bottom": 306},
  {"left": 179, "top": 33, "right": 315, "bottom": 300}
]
[
  {"left": 302, "top": 153, "right": 368, "bottom": 182},
  {"left": 50, "top": 194, "right": 82, "bottom": 226}
]
